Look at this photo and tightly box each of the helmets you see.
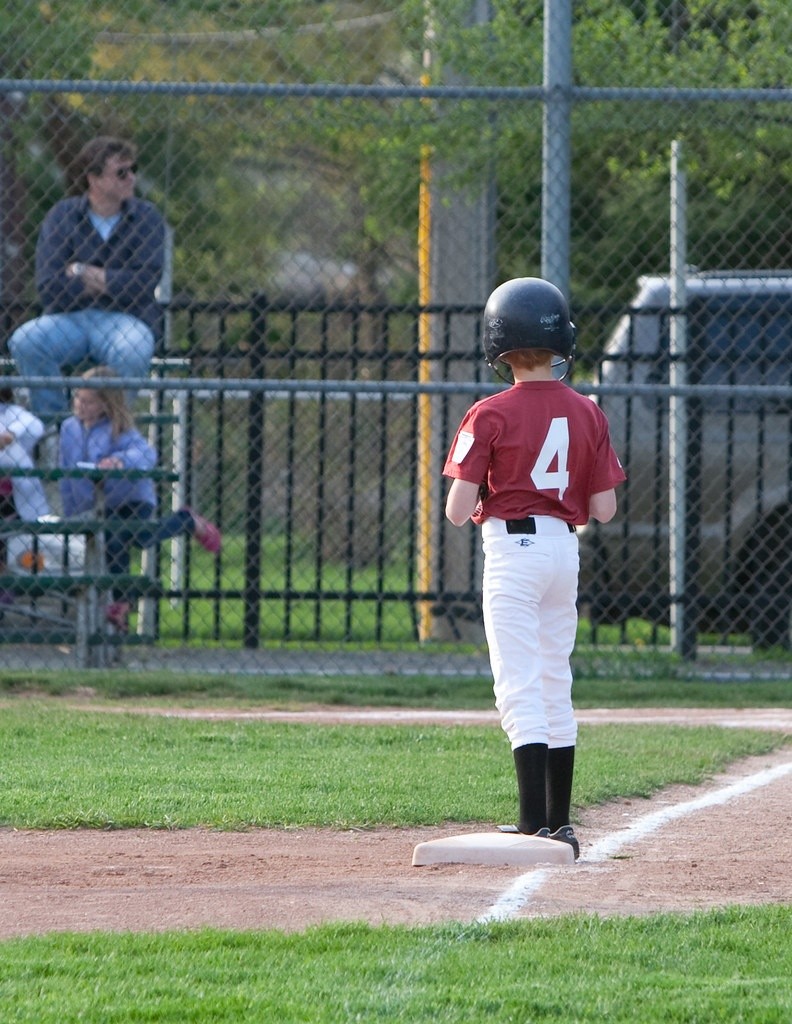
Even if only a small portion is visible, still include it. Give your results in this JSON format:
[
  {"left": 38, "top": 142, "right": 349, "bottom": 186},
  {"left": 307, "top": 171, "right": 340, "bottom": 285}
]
[{"left": 484, "top": 277, "right": 577, "bottom": 384}]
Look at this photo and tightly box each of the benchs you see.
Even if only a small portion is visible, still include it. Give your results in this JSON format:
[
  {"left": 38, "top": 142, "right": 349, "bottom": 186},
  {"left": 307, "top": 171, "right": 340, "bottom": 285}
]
[{"left": 0, "top": 357, "right": 192, "bottom": 669}]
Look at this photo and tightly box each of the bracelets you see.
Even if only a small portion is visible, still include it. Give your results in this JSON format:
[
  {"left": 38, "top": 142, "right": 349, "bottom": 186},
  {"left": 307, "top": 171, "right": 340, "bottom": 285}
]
[{"left": 73, "top": 262, "right": 84, "bottom": 276}]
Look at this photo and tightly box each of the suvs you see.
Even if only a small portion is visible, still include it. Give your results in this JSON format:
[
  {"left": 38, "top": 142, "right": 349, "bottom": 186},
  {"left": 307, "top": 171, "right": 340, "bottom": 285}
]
[{"left": 577, "top": 270, "right": 792, "bottom": 648}]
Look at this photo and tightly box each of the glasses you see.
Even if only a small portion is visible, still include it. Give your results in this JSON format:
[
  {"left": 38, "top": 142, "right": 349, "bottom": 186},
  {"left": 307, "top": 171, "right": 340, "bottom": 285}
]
[{"left": 118, "top": 163, "right": 138, "bottom": 180}]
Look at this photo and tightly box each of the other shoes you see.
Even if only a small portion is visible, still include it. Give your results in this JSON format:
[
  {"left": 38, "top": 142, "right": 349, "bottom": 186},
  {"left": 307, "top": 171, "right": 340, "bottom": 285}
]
[
  {"left": 107, "top": 602, "right": 129, "bottom": 632},
  {"left": 496, "top": 824, "right": 551, "bottom": 839},
  {"left": 548, "top": 825, "right": 579, "bottom": 860},
  {"left": 181, "top": 505, "right": 223, "bottom": 551}
]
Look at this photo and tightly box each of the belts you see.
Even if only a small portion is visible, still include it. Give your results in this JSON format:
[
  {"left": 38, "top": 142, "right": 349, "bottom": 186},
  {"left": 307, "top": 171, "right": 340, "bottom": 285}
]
[{"left": 506, "top": 517, "right": 575, "bottom": 534}]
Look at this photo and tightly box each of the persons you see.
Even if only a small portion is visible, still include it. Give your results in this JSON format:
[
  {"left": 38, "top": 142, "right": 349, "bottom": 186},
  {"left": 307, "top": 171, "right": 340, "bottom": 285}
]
[
  {"left": 59, "top": 367, "right": 224, "bottom": 632},
  {"left": 0, "top": 385, "right": 52, "bottom": 525},
  {"left": 440, "top": 276, "right": 627, "bottom": 860},
  {"left": 9, "top": 137, "right": 174, "bottom": 422}
]
